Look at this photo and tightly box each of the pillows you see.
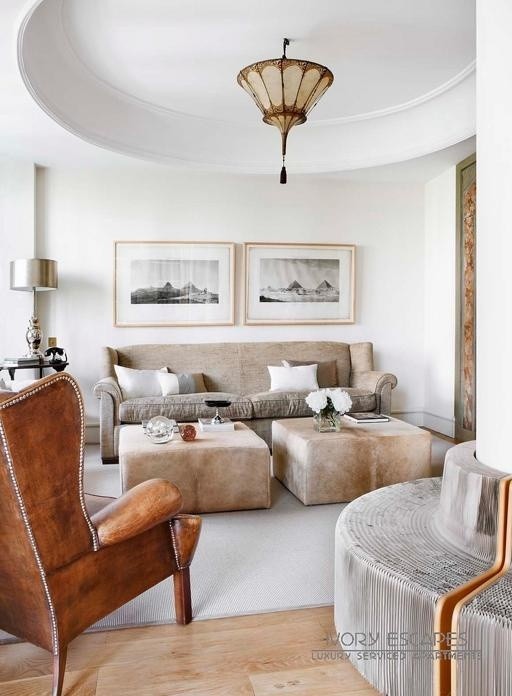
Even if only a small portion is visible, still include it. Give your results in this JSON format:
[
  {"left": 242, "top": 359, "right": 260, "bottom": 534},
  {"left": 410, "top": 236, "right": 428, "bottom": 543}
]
[
  {"left": 267, "top": 364, "right": 319, "bottom": 392},
  {"left": 113, "top": 364, "right": 169, "bottom": 399},
  {"left": 158, "top": 371, "right": 207, "bottom": 396},
  {"left": 282, "top": 359, "right": 339, "bottom": 388}
]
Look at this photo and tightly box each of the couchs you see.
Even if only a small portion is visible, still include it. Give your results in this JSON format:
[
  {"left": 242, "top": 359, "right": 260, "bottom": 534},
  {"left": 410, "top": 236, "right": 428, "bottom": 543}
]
[{"left": 95, "top": 341, "right": 397, "bottom": 464}]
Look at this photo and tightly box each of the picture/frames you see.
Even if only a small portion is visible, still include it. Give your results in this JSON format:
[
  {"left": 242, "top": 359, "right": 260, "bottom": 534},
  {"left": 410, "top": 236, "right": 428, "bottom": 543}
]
[
  {"left": 244, "top": 242, "right": 357, "bottom": 325},
  {"left": 113, "top": 241, "right": 235, "bottom": 327}
]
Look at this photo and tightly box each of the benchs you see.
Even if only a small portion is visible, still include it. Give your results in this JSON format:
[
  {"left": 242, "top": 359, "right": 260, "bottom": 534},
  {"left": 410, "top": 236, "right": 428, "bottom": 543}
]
[
  {"left": 332, "top": 440, "right": 512, "bottom": 696},
  {"left": 449, "top": 479, "right": 511, "bottom": 696}
]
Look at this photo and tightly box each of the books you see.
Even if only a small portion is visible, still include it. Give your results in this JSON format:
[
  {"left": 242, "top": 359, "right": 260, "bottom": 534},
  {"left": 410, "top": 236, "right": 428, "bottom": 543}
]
[
  {"left": 198, "top": 417, "right": 235, "bottom": 433},
  {"left": 3, "top": 356, "right": 40, "bottom": 367},
  {"left": 143, "top": 420, "right": 179, "bottom": 434},
  {"left": 345, "top": 413, "right": 389, "bottom": 424}
]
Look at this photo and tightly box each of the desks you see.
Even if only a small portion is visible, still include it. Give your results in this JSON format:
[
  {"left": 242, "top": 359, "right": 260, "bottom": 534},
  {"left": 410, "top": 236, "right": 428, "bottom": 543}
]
[{"left": 0, "top": 361, "right": 69, "bottom": 379}]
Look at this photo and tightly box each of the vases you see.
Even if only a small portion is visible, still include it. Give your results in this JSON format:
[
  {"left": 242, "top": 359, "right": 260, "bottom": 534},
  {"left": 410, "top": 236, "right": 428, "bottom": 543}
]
[{"left": 314, "top": 412, "right": 341, "bottom": 432}]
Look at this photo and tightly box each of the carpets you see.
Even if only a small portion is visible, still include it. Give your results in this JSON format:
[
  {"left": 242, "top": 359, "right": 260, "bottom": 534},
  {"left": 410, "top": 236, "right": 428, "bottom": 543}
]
[{"left": 0, "top": 427, "right": 458, "bottom": 643}]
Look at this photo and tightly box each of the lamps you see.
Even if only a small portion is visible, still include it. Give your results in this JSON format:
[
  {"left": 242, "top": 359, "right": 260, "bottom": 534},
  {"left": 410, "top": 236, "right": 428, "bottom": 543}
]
[
  {"left": 9, "top": 259, "right": 58, "bottom": 359},
  {"left": 237, "top": 38, "right": 334, "bottom": 184}
]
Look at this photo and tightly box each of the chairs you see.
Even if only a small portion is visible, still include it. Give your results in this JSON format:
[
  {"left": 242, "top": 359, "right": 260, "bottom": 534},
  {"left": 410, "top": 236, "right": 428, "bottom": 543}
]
[{"left": 0, "top": 372, "right": 202, "bottom": 695}]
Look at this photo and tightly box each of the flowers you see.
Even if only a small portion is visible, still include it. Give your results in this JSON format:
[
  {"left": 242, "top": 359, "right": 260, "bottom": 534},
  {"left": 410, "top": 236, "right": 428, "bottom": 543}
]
[{"left": 305, "top": 388, "right": 353, "bottom": 426}]
